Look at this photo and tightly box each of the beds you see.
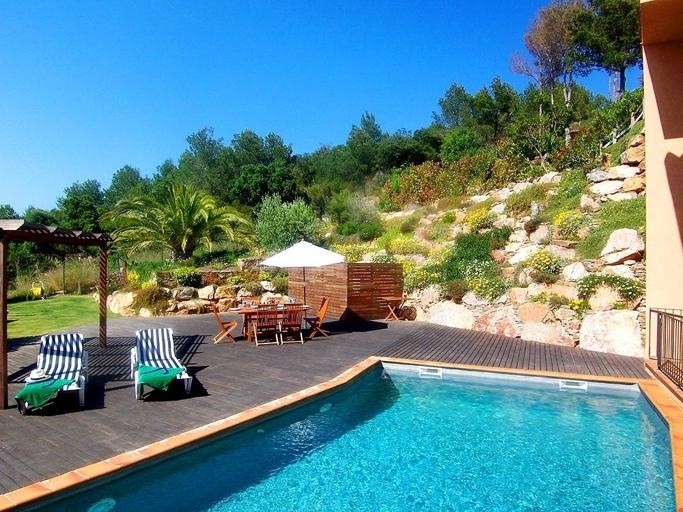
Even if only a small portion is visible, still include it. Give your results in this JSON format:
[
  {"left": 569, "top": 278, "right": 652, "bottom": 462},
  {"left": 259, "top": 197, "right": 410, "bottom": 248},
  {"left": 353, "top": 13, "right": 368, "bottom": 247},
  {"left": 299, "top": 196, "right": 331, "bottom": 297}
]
[{"left": 260, "top": 237, "right": 347, "bottom": 328}]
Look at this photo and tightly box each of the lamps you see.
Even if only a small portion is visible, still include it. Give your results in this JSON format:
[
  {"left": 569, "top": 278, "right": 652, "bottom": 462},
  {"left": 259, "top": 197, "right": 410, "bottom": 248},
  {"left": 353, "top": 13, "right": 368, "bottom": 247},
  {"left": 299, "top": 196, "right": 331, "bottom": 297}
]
[
  {"left": 15, "top": 332, "right": 89, "bottom": 414},
  {"left": 131, "top": 328, "right": 193, "bottom": 400},
  {"left": 211, "top": 296, "right": 334, "bottom": 348}
]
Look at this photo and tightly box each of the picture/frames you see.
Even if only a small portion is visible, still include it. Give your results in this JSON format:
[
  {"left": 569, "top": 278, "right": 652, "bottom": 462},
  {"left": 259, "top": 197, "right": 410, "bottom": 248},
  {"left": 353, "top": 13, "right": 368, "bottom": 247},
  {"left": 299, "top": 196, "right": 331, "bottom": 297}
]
[{"left": 377, "top": 295, "right": 415, "bottom": 321}]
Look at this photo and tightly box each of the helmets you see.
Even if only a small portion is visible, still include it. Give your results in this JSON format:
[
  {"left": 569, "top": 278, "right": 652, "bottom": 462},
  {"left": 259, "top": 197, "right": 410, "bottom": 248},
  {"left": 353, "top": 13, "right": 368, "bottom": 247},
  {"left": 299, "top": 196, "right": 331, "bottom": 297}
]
[
  {"left": 228, "top": 307, "right": 240, "bottom": 311},
  {"left": 303, "top": 305, "right": 310, "bottom": 308},
  {"left": 250, "top": 305, "right": 257, "bottom": 308},
  {"left": 276, "top": 305, "right": 284, "bottom": 308}
]
